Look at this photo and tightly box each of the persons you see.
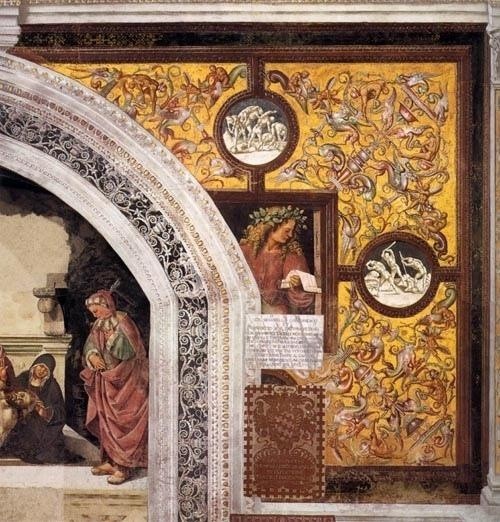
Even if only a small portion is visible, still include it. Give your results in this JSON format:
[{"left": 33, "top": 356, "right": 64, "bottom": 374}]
[
  {"left": 226, "top": 106, "right": 287, "bottom": 152},
  {"left": 366, "top": 249, "right": 429, "bottom": 293},
  {"left": 238, "top": 206, "right": 315, "bottom": 314},
  {"left": 0, "top": 388, "right": 39, "bottom": 447},
  {"left": 85, "top": 291, "right": 148, "bottom": 486},
  {"left": 12, "top": 354, "right": 80, "bottom": 465}
]
